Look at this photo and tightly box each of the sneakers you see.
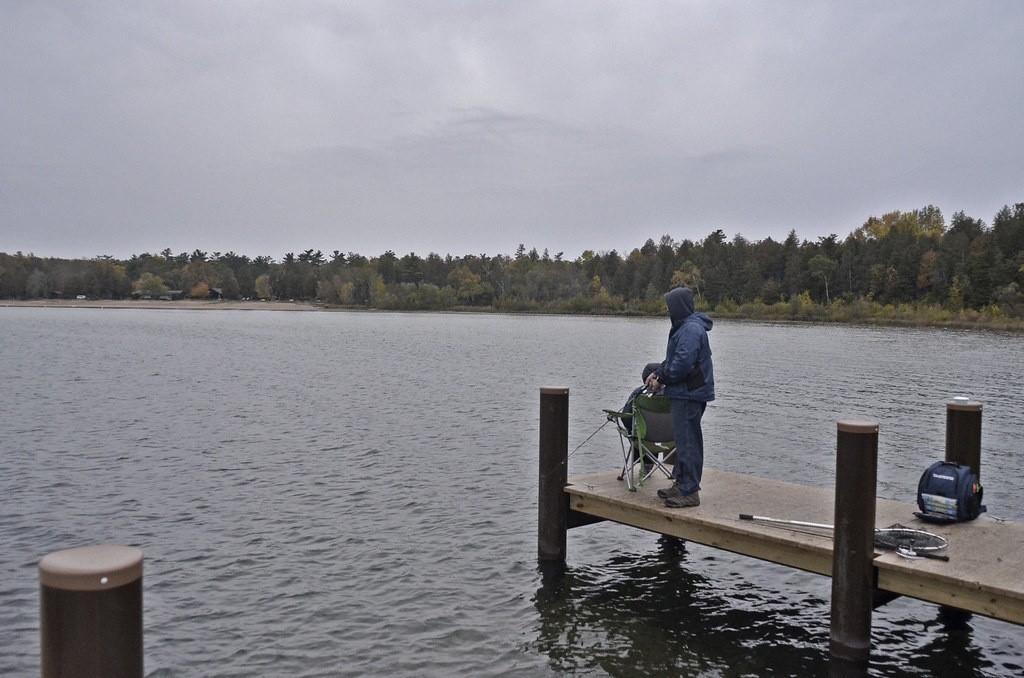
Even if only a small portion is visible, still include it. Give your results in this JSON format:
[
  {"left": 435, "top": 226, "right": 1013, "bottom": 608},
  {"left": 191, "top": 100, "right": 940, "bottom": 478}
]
[{"left": 657, "top": 481, "right": 700, "bottom": 508}]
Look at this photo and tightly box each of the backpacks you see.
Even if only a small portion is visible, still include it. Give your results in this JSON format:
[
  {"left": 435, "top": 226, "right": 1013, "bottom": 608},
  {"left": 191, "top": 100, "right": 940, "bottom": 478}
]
[{"left": 913, "top": 459, "right": 988, "bottom": 524}]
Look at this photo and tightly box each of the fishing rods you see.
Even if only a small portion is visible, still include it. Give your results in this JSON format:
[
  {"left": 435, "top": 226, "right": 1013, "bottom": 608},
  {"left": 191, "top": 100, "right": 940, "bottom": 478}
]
[{"left": 516, "top": 373, "right": 662, "bottom": 503}]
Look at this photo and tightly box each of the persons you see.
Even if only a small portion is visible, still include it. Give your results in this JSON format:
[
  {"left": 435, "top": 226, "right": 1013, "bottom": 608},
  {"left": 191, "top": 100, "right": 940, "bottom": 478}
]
[
  {"left": 646, "top": 287, "right": 716, "bottom": 508},
  {"left": 621, "top": 363, "right": 665, "bottom": 478}
]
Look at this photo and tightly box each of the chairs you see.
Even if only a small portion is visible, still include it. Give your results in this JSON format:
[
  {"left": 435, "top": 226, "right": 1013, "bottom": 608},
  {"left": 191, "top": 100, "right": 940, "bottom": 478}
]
[{"left": 602, "top": 392, "right": 677, "bottom": 491}]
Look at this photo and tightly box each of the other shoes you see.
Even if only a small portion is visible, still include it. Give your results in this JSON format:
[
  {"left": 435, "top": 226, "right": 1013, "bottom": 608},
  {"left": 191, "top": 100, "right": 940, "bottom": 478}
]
[{"left": 640, "top": 463, "right": 653, "bottom": 478}]
